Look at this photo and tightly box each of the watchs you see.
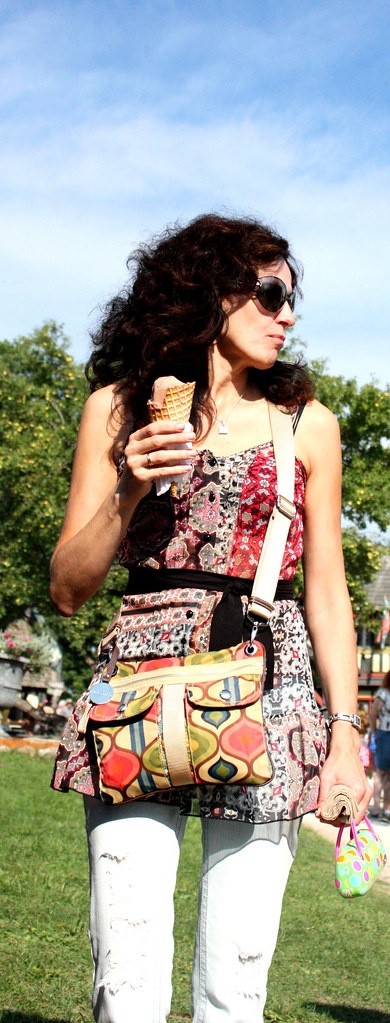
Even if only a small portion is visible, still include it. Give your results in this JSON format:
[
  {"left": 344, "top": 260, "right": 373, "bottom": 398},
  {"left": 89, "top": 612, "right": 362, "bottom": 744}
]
[{"left": 329, "top": 712, "right": 361, "bottom": 731}]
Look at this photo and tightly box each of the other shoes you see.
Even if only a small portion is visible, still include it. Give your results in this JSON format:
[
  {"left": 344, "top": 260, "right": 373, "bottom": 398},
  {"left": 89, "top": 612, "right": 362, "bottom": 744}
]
[
  {"left": 371, "top": 814, "right": 379, "bottom": 817},
  {"left": 381, "top": 812, "right": 390, "bottom": 823}
]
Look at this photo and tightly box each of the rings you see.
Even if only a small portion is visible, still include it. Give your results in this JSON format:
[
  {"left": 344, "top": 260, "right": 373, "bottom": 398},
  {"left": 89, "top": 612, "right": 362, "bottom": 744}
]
[
  {"left": 148, "top": 453, "right": 154, "bottom": 466},
  {"left": 364, "top": 809, "right": 370, "bottom": 816}
]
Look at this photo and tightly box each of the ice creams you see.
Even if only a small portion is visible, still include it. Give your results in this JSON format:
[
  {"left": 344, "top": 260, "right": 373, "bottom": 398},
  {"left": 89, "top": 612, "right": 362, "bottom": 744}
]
[{"left": 147, "top": 374, "right": 196, "bottom": 425}]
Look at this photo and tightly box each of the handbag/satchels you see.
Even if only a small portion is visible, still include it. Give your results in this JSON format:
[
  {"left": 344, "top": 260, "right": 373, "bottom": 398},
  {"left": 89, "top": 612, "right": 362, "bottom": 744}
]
[
  {"left": 333, "top": 814, "right": 385, "bottom": 897},
  {"left": 88, "top": 640, "right": 273, "bottom": 801}
]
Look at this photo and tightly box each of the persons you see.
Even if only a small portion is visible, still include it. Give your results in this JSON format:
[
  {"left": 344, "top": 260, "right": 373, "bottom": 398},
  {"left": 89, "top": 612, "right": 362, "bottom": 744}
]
[
  {"left": 6, "top": 698, "right": 72, "bottom": 736},
  {"left": 49, "top": 214, "right": 375, "bottom": 1023},
  {"left": 359, "top": 670, "right": 390, "bottom": 820}
]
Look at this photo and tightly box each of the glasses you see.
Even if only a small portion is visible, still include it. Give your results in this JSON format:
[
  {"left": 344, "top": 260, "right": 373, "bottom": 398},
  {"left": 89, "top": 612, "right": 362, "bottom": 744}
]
[{"left": 244, "top": 276, "right": 296, "bottom": 312}]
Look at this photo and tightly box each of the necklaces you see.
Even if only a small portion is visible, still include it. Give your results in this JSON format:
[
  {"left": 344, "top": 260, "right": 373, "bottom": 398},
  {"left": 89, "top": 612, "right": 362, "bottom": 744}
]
[{"left": 213, "top": 391, "right": 247, "bottom": 434}]
